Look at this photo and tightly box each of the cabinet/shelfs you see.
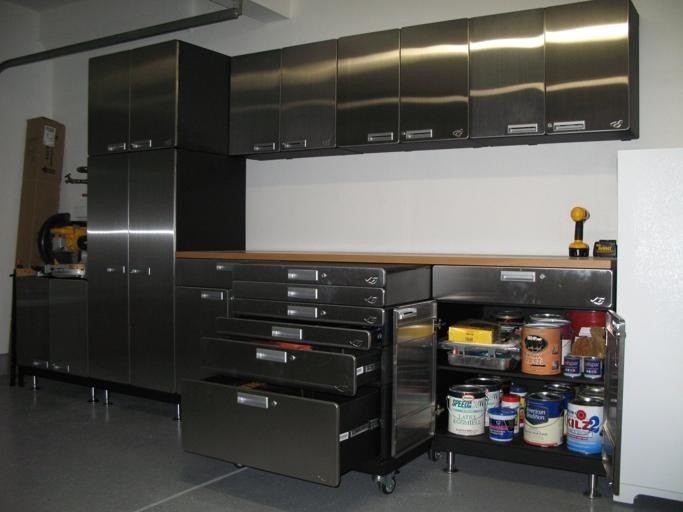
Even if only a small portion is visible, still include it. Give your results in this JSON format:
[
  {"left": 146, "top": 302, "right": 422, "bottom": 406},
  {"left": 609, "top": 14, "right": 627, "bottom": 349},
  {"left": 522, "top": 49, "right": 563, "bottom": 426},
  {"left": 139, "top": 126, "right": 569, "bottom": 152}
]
[
  {"left": 86, "top": 148, "right": 246, "bottom": 420},
  {"left": 469, "top": 0, "right": 640, "bottom": 148},
  {"left": 390, "top": 300, "right": 626, "bottom": 501},
  {"left": 171, "top": 250, "right": 432, "bottom": 496},
  {"left": 227, "top": 38, "right": 336, "bottom": 160},
  {"left": 335, "top": 18, "right": 469, "bottom": 155},
  {"left": 86, "top": 39, "right": 228, "bottom": 156},
  {"left": 10, "top": 277, "right": 88, "bottom": 391}
]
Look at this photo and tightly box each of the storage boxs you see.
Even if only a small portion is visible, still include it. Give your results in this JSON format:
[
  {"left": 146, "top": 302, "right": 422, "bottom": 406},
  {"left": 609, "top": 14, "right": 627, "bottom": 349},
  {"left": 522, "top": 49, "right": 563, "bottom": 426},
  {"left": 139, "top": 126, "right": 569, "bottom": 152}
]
[{"left": 14, "top": 116, "right": 65, "bottom": 278}]
